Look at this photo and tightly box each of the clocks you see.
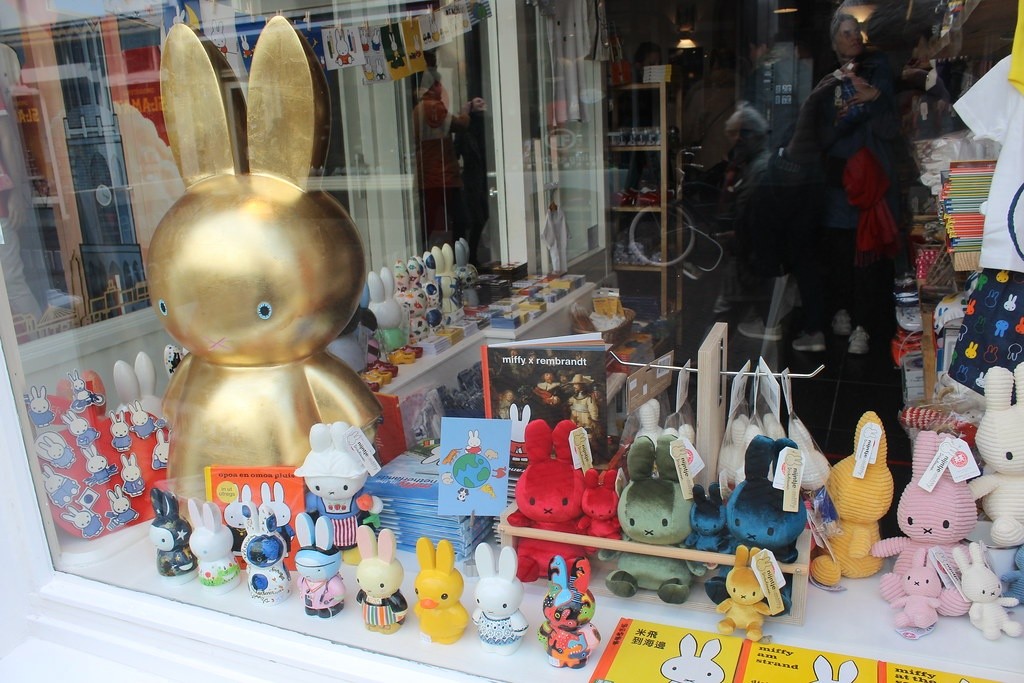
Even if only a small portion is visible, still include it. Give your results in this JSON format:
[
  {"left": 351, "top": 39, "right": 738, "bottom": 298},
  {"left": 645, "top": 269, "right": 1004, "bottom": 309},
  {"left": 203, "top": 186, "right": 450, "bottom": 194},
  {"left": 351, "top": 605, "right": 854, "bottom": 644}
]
[{"left": 96, "top": 185, "right": 112, "bottom": 206}]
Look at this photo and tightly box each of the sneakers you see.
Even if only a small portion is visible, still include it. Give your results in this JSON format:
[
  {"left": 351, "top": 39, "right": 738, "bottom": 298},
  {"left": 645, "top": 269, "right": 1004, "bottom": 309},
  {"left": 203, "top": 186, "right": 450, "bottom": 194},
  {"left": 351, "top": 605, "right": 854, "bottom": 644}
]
[
  {"left": 737, "top": 318, "right": 782, "bottom": 341},
  {"left": 792, "top": 332, "right": 826, "bottom": 351},
  {"left": 848, "top": 327, "right": 870, "bottom": 353},
  {"left": 832, "top": 309, "right": 852, "bottom": 334}
]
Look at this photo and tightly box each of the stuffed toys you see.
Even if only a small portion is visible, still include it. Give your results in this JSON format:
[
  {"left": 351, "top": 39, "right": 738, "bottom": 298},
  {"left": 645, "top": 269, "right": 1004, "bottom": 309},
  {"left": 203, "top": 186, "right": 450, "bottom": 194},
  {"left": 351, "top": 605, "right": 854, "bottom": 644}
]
[
  {"left": 703, "top": 436, "right": 807, "bottom": 617},
  {"left": 716, "top": 544, "right": 773, "bottom": 640},
  {"left": 968, "top": 362, "right": 1024, "bottom": 545},
  {"left": 892, "top": 547, "right": 940, "bottom": 628},
  {"left": 508, "top": 418, "right": 587, "bottom": 578},
  {"left": 598, "top": 436, "right": 707, "bottom": 603},
  {"left": 1002, "top": 544, "right": 1024, "bottom": 604},
  {"left": 871, "top": 430, "right": 979, "bottom": 616},
  {"left": 294, "top": 422, "right": 383, "bottom": 566},
  {"left": 686, "top": 482, "right": 730, "bottom": 554},
  {"left": 578, "top": 469, "right": 621, "bottom": 552},
  {"left": 810, "top": 411, "right": 894, "bottom": 587},
  {"left": 953, "top": 541, "right": 1024, "bottom": 640}
]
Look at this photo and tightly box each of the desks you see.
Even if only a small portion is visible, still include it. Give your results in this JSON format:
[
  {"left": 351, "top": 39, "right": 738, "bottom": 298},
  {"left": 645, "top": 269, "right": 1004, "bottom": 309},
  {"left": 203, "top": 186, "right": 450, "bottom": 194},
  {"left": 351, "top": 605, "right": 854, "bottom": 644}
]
[{"left": 376, "top": 274, "right": 597, "bottom": 404}]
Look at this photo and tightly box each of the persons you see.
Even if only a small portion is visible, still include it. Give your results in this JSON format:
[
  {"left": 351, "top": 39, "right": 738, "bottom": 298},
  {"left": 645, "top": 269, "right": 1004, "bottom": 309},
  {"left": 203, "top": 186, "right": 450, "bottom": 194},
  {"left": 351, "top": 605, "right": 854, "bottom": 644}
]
[
  {"left": 624, "top": 41, "right": 738, "bottom": 200},
  {"left": 716, "top": 186, "right": 876, "bottom": 353},
  {"left": 788, "top": 46, "right": 903, "bottom": 229},
  {"left": 415, "top": 69, "right": 489, "bottom": 266},
  {"left": 829, "top": 13, "right": 864, "bottom": 63},
  {"left": 727, "top": 107, "right": 772, "bottom": 177}
]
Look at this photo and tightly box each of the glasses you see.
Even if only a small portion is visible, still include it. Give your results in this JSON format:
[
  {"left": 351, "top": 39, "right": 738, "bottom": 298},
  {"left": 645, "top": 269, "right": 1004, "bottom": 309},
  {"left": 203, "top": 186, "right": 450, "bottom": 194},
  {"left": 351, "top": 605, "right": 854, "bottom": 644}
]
[{"left": 725, "top": 128, "right": 762, "bottom": 138}]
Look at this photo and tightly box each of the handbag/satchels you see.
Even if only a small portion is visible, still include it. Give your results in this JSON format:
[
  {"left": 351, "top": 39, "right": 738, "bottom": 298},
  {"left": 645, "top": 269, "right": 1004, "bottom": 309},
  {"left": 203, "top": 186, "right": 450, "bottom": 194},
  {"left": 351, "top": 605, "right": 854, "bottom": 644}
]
[{"left": 682, "top": 142, "right": 700, "bottom": 166}]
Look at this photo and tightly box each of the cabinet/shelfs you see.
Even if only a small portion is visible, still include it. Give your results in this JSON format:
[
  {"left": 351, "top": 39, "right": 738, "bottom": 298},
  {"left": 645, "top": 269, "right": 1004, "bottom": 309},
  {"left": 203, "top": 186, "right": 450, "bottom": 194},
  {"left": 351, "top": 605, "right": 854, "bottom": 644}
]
[{"left": 605, "top": 76, "right": 685, "bottom": 346}]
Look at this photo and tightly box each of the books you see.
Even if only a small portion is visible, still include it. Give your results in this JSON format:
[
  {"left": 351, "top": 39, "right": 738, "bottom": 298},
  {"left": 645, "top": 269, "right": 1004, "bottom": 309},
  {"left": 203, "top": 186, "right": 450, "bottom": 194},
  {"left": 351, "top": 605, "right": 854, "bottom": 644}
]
[{"left": 481, "top": 332, "right": 608, "bottom": 463}]
